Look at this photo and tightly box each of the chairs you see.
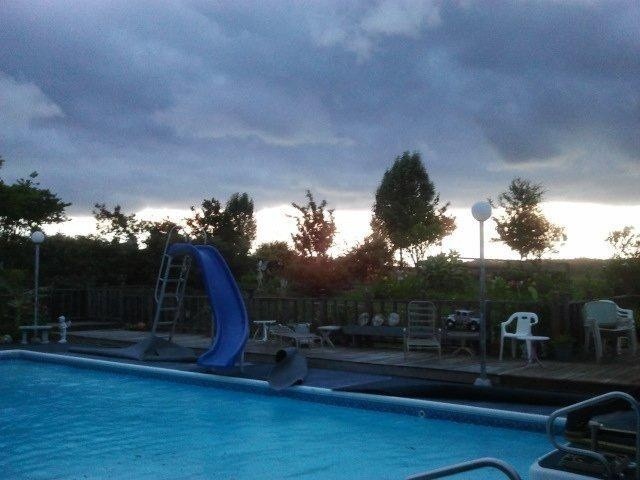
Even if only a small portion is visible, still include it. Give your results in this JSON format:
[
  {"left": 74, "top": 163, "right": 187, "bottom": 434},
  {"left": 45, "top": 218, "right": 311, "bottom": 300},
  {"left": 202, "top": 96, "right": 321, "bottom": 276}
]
[
  {"left": 498, "top": 311, "right": 539, "bottom": 360},
  {"left": 581, "top": 299, "right": 636, "bottom": 366},
  {"left": 269, "top": 325, "right": 320, "bottom": 351},
  {"left": 403, "top": 301, "right": 442, "bottom": 358}
]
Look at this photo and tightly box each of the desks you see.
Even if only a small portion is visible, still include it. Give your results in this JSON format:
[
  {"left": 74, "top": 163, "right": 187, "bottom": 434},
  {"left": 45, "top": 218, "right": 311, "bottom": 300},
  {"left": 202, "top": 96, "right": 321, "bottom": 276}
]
[
  {"left": 516, "top": 336, "right": 550, "bottom": 368},
  {"left": 317, "top": 326, "right": 339, "bottom": 351},
  {"left": 253, "top": 320, "right": 276, "bottom": 341}
]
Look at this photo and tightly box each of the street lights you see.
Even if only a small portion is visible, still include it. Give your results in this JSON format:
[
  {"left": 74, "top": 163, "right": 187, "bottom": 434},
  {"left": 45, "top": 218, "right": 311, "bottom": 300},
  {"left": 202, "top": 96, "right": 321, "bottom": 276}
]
[
  {"left": 29, "top": 229, "right": 46, "bottom": 342},
  {"left": 472, "top": 200, "right": 495, "bottom": 387}
]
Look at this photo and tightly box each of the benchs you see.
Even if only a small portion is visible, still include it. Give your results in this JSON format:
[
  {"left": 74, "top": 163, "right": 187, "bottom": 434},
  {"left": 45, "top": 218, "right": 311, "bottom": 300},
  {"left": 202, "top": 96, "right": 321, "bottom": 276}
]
[{"left": 343, "top": 323, "right": 479, "bottom": 355}]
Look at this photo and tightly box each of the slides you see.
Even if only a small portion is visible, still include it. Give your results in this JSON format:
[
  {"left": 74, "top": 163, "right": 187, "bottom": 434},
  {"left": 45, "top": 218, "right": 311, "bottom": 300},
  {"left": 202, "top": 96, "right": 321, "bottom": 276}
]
[{"left": 167, "top": 242, "right": 250, "bottom": 369}]
